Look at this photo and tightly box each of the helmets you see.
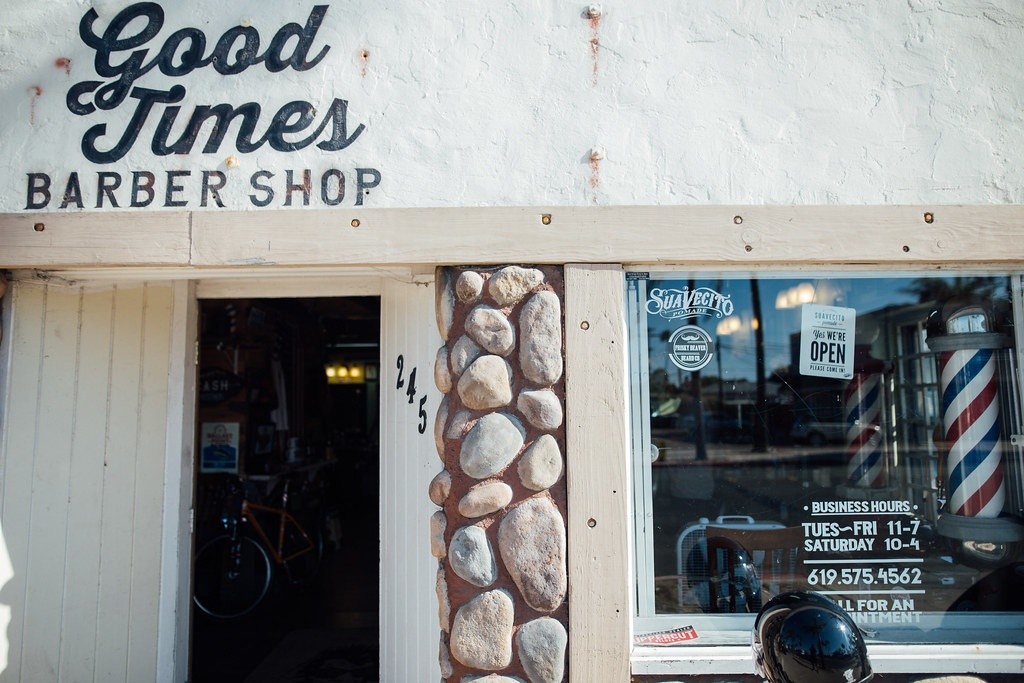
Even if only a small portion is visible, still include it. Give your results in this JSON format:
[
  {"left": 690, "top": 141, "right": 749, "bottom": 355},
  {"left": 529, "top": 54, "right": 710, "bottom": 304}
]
[
  {"left": 686, "top": 536, "right": 763, "bottom": 614},
  {"left": 752, "top": 591, "right": 874, "bottom": 683}
]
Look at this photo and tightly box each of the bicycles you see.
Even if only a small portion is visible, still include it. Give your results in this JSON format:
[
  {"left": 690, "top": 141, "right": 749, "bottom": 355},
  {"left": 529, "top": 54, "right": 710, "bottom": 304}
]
[{"left": 192, "top": 469, "right": 325, "bottom": 622}]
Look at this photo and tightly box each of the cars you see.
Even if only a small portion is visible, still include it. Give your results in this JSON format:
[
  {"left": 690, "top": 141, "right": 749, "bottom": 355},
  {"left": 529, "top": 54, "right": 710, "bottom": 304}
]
[{"left": 792, "top": 405, "right": 883, "bottom": 449}]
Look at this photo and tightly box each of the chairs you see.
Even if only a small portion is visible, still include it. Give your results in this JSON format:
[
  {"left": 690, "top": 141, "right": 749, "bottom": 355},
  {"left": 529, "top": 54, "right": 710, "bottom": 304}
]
[{"left": 706, "top": 522, "right": 814, "bottom": 614}]
